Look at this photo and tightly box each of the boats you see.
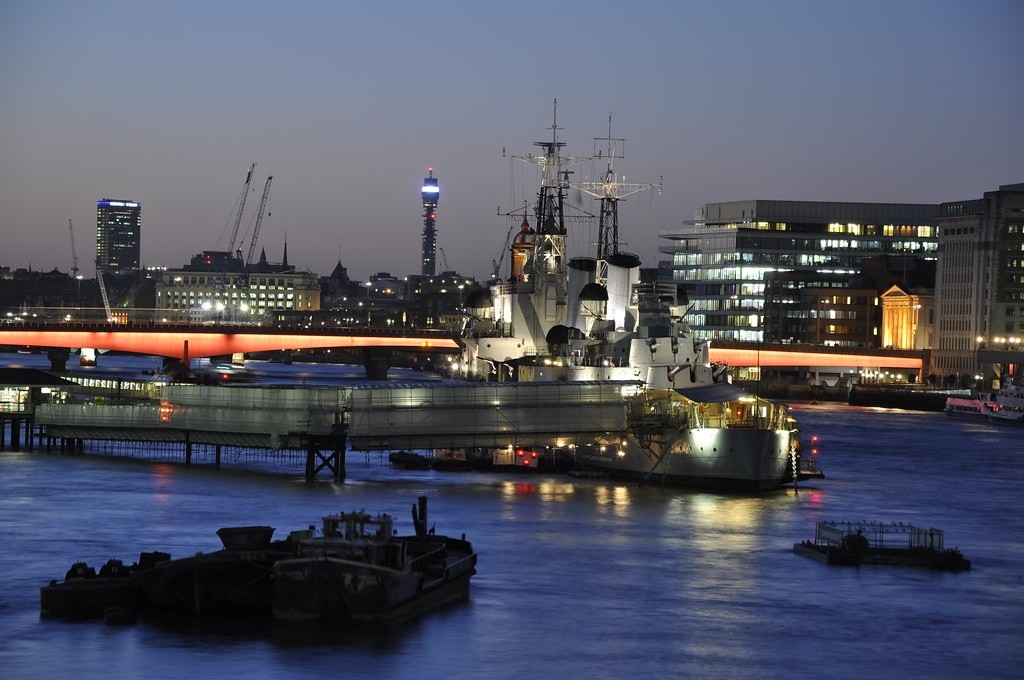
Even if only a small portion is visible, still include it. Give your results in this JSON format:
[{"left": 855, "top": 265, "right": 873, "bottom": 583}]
[
  {"left": 945, "top": 375, "right": 1024, "bottom": 426},
  {"left": 37, "top": 495, "right": 479, "bottom": 636},
  {"left": 793, "top": 520, "right": 972, "bottom": 570},
  {"left": 387, "top": 93, "right": 798, "bottom": 495}
]
